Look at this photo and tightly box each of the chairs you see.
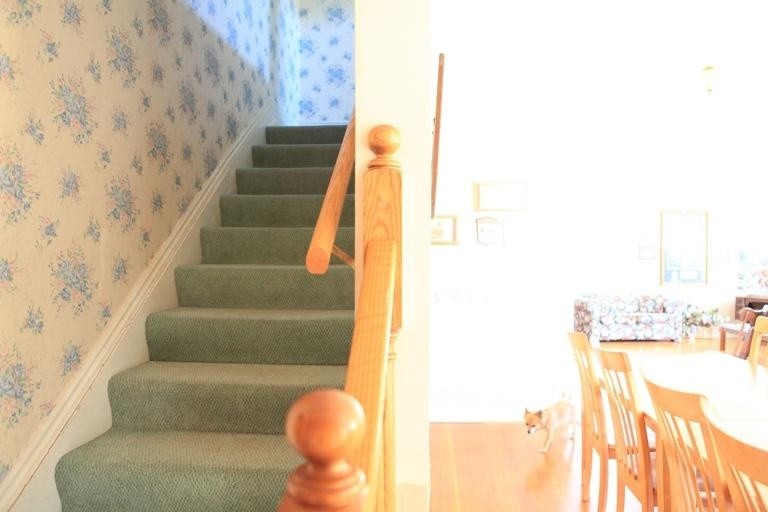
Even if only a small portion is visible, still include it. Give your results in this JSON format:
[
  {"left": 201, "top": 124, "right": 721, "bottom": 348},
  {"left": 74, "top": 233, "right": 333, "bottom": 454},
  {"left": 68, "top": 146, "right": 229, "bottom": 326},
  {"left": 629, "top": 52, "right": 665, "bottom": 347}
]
[
  {"left": 565, "top": 315, "right": 768, "bottom": 493},
  {"left": 719, "top": 304, "right": 767, "bottom": 355}
]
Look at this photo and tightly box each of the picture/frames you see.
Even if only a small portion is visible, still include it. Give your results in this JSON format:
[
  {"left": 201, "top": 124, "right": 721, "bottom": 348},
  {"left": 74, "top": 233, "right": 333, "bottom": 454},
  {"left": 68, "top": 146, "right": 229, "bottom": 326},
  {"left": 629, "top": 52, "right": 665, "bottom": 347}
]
[
  {"left": 430, "top": 213, "right": 459, "bottom": 248},
  {"left": 472, "top": 179, "right": 533, "bottom": 214},
  {"left": 657, "top": 206, "right": 712, "bottom": 287}
]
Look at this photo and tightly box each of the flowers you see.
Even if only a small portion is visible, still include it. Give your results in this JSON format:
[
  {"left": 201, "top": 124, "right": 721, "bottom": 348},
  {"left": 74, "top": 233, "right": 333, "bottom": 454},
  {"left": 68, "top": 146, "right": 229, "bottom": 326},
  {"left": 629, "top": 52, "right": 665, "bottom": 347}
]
[{"left": 690, "top": 309, "right": 721, "bottom": 330}]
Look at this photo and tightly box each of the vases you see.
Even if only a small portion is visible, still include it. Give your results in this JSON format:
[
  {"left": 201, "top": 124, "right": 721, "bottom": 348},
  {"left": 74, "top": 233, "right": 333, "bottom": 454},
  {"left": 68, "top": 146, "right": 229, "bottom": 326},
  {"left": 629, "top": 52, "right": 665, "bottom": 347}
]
[{"left": 696, "top": 327, "right": 716, "bottom": 340}]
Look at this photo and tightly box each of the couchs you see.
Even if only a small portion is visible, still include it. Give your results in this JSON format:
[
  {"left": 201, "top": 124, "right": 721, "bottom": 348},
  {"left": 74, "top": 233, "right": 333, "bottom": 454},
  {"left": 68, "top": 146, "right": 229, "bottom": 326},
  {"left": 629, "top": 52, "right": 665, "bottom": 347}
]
[{"left": 573, "top": 292, "right": 686, "bottom": 348}]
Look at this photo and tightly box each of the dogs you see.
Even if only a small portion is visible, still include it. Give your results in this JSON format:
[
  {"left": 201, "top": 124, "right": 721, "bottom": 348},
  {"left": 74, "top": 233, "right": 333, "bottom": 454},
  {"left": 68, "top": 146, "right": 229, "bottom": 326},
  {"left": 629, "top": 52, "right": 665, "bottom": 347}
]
[{"left": 524, "top": 397, "right": 578, "bottom": 453}]
